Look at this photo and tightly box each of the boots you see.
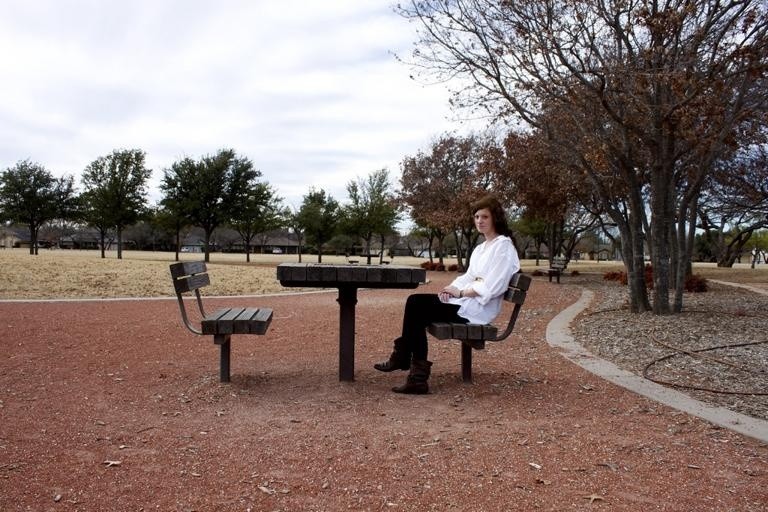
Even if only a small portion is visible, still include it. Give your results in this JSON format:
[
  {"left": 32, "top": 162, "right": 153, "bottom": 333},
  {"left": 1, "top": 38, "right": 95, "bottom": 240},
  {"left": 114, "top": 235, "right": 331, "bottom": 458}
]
[
  {"left": 392, "top": 359, "right": 432, "bottom": 394},
  {"left": 374, "top": 336, "right": 412, "bottom": 373}
]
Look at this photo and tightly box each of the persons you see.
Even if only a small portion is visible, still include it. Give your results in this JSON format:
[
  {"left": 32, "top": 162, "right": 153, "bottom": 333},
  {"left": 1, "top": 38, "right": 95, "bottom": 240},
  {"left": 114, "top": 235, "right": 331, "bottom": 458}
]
[{"left": 371, "top": 196, "right": 524, "bottom": 396}]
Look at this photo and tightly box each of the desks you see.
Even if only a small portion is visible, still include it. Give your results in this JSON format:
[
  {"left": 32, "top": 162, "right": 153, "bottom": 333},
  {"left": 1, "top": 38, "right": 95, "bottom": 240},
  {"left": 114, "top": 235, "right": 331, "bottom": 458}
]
[{"left": 277, "top": 263, "right": 426, "bottom": 382}]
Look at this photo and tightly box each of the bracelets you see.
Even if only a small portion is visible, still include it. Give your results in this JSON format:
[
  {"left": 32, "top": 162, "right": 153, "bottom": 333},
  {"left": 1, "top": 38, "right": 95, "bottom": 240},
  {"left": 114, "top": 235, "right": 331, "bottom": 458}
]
[{"left": 459, "top": 289, "right": 464, "bottom": 298}]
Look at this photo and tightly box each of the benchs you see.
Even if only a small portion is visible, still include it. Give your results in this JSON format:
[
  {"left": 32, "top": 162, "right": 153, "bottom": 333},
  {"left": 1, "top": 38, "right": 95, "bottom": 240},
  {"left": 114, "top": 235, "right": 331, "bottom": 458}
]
[
  {"left": 169, "top": 260, "right": 273, "bottom": 383},
  {"left": 536, "top": 256, "right": 570, "bottom": 283},
  {"left": 428, "top": 273, "right": 532, "bottom": 384}
]
